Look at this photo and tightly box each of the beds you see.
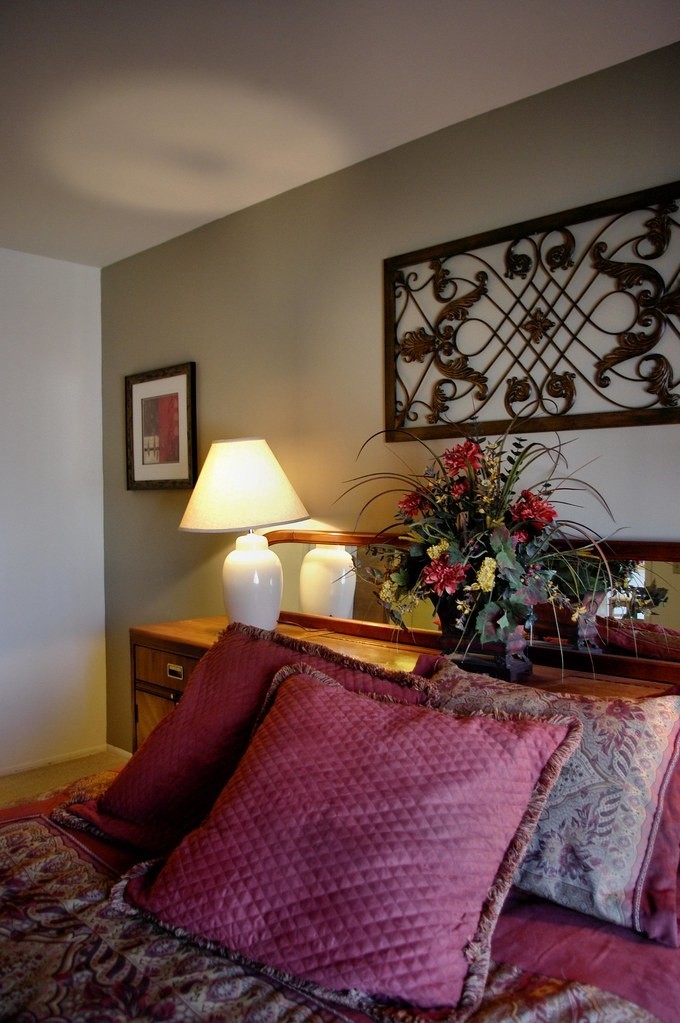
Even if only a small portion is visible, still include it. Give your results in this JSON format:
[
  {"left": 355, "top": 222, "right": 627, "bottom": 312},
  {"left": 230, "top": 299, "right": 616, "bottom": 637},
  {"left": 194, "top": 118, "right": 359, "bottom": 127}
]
[{"left": 0, "top": 620, "right": 680, "bottom": 1023}]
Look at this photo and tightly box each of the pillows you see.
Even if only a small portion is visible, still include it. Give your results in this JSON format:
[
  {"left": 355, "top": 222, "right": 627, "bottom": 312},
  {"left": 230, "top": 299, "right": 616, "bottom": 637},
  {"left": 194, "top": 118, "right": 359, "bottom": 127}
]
[
  {"left": 109, "top": 663, "right": 584, "bottom": 1023},
  {"left": 414, "top": 652, "right": 680, "bottom": 948},
  {"left": 95, "top": 616, "right": 438, "bottom": 841}
]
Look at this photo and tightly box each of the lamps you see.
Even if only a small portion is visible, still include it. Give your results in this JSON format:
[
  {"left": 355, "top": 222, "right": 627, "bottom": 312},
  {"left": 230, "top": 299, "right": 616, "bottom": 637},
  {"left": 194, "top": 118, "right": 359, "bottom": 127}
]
[
  {"left": 179, "top": 437, "right": 309, "bottom": 630},
  {"left": 299, "top": 542, "right": 357, "bottom": 619}
]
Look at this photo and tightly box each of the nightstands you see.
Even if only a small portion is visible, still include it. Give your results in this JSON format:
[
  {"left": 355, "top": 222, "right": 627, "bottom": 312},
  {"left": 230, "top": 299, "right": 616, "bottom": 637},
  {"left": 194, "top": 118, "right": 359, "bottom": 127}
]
[{"left": 130, "top": 617, "right": 306, "bottom": 759}]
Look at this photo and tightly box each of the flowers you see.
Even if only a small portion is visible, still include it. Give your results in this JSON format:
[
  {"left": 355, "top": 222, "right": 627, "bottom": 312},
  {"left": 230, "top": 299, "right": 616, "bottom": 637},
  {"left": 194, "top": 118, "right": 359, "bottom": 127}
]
[{"left": 330, "top": 394, "right": 675, "bottom": 682}]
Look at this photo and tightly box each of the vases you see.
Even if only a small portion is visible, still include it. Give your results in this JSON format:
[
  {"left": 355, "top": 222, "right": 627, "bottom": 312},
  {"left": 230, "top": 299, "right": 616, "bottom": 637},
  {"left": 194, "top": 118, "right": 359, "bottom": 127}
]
[{"left": 425, "top": 590, "right": 507, "bottom": 653}]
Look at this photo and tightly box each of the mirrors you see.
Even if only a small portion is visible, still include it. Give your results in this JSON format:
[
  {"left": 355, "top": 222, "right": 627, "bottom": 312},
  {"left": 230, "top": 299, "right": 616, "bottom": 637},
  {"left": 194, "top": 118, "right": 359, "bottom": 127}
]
[{"left": 267, "top": 542, "right": 680, "bottom": 664}]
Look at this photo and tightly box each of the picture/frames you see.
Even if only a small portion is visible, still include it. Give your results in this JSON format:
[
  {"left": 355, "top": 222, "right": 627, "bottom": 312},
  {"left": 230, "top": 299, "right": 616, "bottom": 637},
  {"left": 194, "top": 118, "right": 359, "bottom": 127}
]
[{"left": 125, "top": 361, "right": 197, "bottom": 491}]
